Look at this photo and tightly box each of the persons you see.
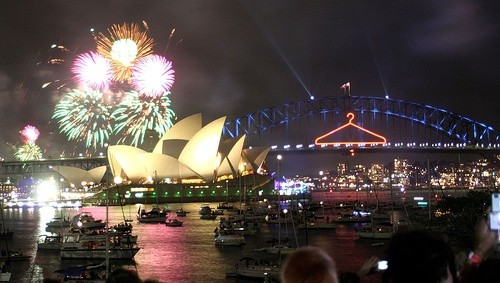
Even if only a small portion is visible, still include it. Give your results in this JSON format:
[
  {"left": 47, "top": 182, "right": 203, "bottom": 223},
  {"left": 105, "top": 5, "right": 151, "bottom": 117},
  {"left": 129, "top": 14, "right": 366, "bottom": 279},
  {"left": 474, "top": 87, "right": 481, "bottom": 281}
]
[
  {"left": 382, "top": 224, "right": 458, "bottom": 283},
  {"left": 281, "top": 246, "right": 378, "bottom": 283}
]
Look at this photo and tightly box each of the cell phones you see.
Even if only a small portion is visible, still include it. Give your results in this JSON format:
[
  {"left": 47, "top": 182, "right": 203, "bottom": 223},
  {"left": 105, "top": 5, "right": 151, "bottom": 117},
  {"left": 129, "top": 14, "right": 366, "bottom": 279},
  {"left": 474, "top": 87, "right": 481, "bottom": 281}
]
[
  {"left": 491, "top": 193, "right": 499, "bottom": 212},
  {"left": 489, "top": 213, "right": 500, "bottom": 229},
  {"left": 376, "top": 259, "right": 389, "bottom": 271}
]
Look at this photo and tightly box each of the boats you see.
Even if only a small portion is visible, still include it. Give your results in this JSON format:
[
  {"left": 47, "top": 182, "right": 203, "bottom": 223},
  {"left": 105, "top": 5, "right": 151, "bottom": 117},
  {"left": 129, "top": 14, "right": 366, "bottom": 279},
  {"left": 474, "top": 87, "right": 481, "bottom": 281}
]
[
  {"left": 197, "top": 190, "right": 433, "bottom": 283},
  {"left": 165, "top": 219, "right": 183, "bottom": 226},
  {"left": 79, "top": 222, "right": 138, "bottom": 242},
  {"left": 36, "top": 232, "right": 113, "bottom": 251},
  {"left": 0, "top": 229, "right": 14, "bottom": 239},
  {"left": 55, "top": 262, "right": 116, "bottom": 281},
  {"left": 159, "top": 208, "right": 171, "bottom": 213},
  {"left": 70, "top": 213, "right": 105, "bottom": 230},
  {"left": 137, "top": 212, "right": 167, "bottom": 224},
  {"left": 60, "top": 240, "right": 141, "bottom": 260},
  {"left": 150, "top": 206, "right": 160, "bottom": 212},
  {"left": 0, "top": 251, "right": 33, "bottom": 261},
  {"left": 46, "top": 217, "right": 70, "bottom": 227},
  {"left": 175, "top": 208, "right": 186, "bottom": 216}
]
